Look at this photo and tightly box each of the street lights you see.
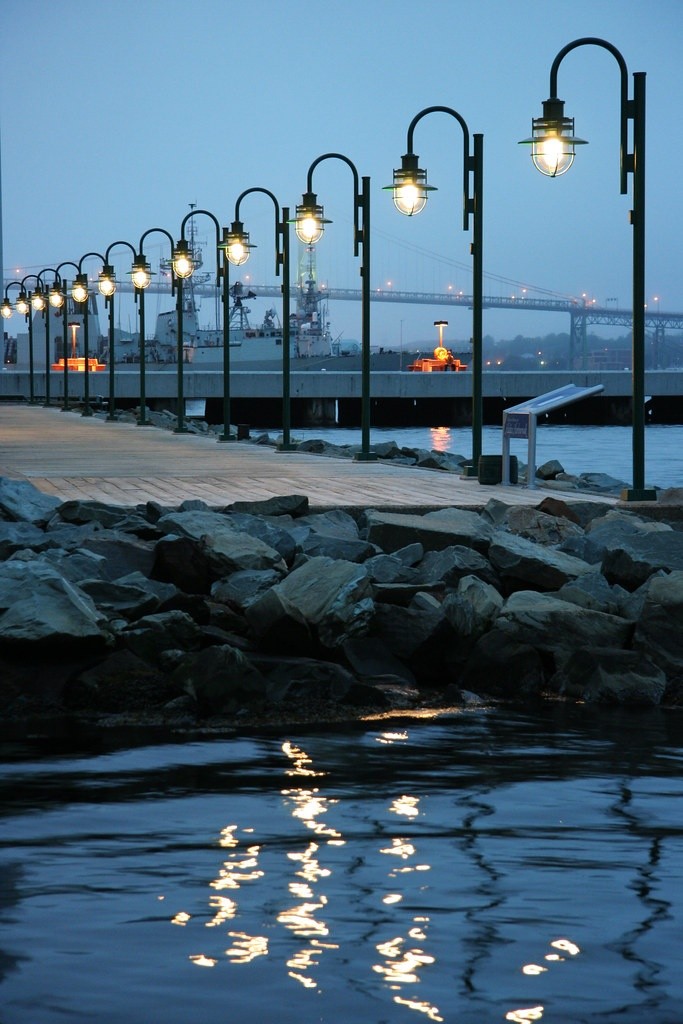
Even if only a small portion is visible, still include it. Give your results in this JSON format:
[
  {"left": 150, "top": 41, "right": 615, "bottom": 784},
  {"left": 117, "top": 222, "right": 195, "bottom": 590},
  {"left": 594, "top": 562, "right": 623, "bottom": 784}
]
[
  {"left": 164, "top": 208, "right": 236, "bottom": 441},
  {"left": 92, "top": 241, "right": 151, "bottom": 426},
  {"left": 123, "top": 226, "right": 189, "bottom": 436},
  {"left": 0, "top": 281, "right": 38, "bottom": 406},
  {"left": 433, "top": 320, "right": 449, "bottom": 361},
  {"left": 43, "top": 261, "right": 93, "bottom": 417},
  {"left": 25, "top": 268, "right": 72, "bottom": 412},
  {"left": 519, "top": 36, "right": 658, "bottom": 500},
  {"left": 67, "top": 321, "right": 80, "bottom": 358},
  {"left": 286, "top": 151, "right": 378, "bottom": 462},
  {"left": 380, "top": 105, "right": 486, "bottom": 478},
  {"left": 12, "top": 275, "right": 53, "bottom": 409},
  {"left": 64, "top": 252, "right": 120, "bottom": 424},
  {"left": 218, "top": 186, "right": 298, "bottom": 454}
]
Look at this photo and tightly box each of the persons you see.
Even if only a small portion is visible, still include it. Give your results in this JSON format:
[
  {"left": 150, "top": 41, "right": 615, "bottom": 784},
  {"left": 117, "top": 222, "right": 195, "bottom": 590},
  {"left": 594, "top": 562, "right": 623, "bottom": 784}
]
[{"left": 444, "top": 351, "right": 455, "bottom": 371}]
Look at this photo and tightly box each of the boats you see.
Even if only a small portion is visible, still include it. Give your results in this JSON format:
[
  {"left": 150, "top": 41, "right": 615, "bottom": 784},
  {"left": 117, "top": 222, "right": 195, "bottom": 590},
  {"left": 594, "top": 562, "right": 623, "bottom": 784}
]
[{"left": 0, "top": 198, "right": 475, "bottom": 429}]
